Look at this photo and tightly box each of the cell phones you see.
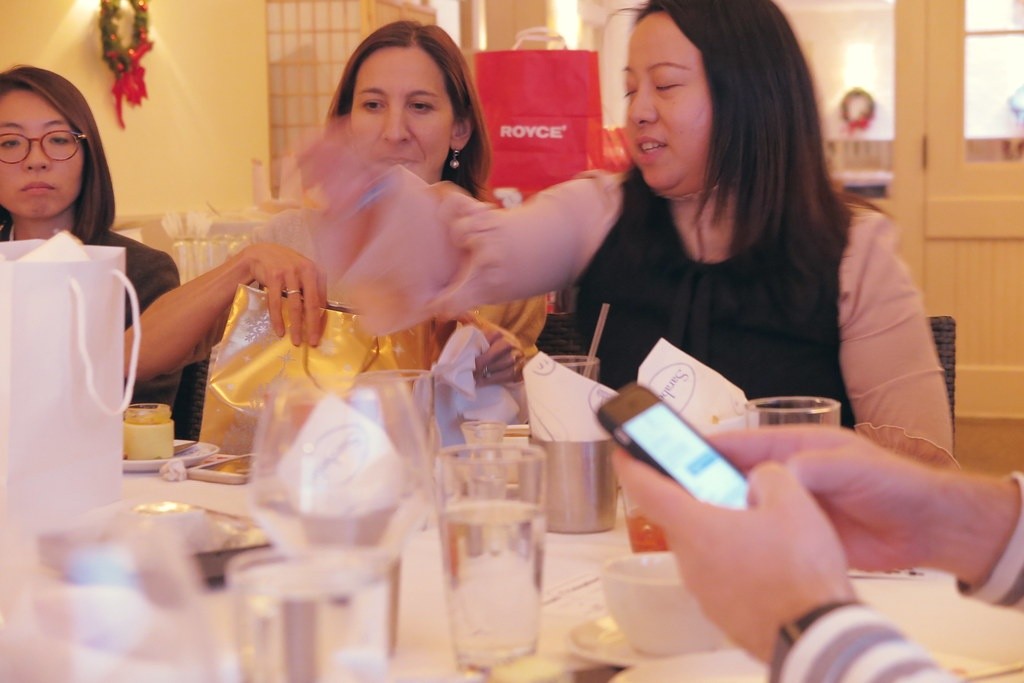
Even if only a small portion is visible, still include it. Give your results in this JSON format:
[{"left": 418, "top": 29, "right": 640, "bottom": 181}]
[
  {"left": 188, "top": 453, "right": 253, "bottom": 486},
  {"left": 595, "top": 385, "right": 750, "bottom": 509}
]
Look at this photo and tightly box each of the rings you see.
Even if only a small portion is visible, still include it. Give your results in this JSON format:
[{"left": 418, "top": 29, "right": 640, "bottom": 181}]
[{"left": 288, "top": 290, "right": 302, "bottom": 294}]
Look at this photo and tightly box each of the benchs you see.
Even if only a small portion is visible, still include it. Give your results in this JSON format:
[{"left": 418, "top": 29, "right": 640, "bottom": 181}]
[{"left": 166, "top": 312, "right": 961, "bottom": 447}]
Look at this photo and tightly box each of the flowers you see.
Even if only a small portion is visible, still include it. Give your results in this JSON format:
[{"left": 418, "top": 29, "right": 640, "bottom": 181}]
[{"left": 99, "top": 0, "right": 156, "bottom": 129}]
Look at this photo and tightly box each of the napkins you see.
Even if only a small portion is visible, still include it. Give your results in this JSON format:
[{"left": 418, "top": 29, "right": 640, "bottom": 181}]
[
  {"left": 274, "top": 386, "right": 400, "bottom": 509},
  {"left": 633, "top": 338, "right": 755, "bottom": 436},
  {"left": 523, "top": 348, "right": 619, "bottom": 442}
]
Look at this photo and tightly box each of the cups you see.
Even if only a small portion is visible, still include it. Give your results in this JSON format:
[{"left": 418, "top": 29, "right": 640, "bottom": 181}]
[
  {"left": 616, "top": 472, "right": 669, "bottom": 554},
  {"left": 549, "top": 355, "right": 600, "bottom": 383},
  {"left": 223, "top": 546, "right": 401, "bottom": 683},
  {"left": 436, "top": 443, "right": 547, "bottom": 667},
  {"left": 744, "top": 396, "right": 841, "bottom": 428},
  {"left": 356, "top": 369, "right": 434, "bottom": 469},
  {"left": 248, "top": 377, "right": 429, "bottom": 553},
  {"left": 599, "top": 551, "right": 722, "bottom": 656}
]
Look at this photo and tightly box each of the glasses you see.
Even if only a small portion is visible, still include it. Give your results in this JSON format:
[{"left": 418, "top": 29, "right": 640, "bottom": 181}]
[{"left": 0, "top": 130, "right": 86, "bottom": 164}]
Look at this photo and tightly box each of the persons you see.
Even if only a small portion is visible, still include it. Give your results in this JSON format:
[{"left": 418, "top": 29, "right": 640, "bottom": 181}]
[
  {"left": 119, "top": 18, "right": 550, "bottom": 425},
  {"left": 288, "top": 0, "right": 965, "bottom": 469},
  {"left": 612, "top": 424, "right": 1024, "bottom": 682},
  {"left": 0, "top": 63, "right": 184, "bottom": 409}
]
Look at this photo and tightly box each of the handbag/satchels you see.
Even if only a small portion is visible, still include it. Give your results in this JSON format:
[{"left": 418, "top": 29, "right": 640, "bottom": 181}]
[
  {"left": 198, "top": 284, "right": 458, "bottom": 475},
  {"left": 0, "top": 239, "right": 140, "bottom": 530},
  {"left": 470, "top": 26, "right": 606, "bottom": 209}
]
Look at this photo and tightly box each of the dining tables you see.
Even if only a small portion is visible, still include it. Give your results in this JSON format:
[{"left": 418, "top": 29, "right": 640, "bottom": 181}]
[{"left": 0, "top": 433, "right": 1024, "bottom": 683}]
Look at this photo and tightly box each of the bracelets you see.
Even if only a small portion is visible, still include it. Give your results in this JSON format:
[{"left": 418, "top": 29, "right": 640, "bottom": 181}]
[{"left": 769, "top": 599, "right": 856, "bottom": 682}]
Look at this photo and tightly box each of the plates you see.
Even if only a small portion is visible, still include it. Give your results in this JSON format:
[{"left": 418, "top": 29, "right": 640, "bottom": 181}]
[
  {"left": 562, "top": 614, "right": 649, "bottom": 670},
  {"left": 123, "top": 441, "right": 220, "bottom": 473}
]
